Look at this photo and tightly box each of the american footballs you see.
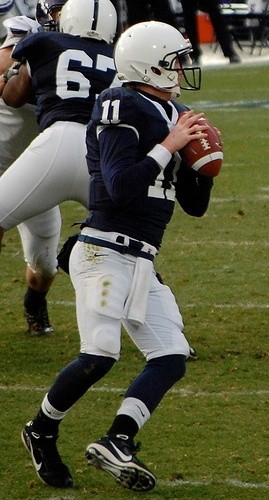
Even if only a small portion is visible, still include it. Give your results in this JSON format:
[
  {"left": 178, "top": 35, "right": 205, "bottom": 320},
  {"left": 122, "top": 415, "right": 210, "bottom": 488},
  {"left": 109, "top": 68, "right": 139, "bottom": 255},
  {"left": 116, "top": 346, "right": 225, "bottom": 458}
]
[{"left": 176, "top": 110, "right": 223, "bottom": 176}]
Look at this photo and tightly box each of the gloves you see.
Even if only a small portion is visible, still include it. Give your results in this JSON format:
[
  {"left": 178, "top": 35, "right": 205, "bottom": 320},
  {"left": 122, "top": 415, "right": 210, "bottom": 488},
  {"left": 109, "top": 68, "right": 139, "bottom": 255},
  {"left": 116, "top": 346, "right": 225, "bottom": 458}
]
[{"left": 3, "top": 62, "right": 22, "bottom": 84}]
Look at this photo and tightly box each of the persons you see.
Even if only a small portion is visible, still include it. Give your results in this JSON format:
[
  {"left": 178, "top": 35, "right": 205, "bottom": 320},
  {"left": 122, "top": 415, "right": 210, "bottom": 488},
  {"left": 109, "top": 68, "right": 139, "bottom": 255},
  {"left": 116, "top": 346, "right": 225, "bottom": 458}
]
[
  {"left": 0, "top": 0, "right": 126, "bottom": 338},
  {"left": 21, "top": 21, "right": 224, "bottom": 493},
  {"left": 126, "top": 0, "right": 241, "bottom": 65}
]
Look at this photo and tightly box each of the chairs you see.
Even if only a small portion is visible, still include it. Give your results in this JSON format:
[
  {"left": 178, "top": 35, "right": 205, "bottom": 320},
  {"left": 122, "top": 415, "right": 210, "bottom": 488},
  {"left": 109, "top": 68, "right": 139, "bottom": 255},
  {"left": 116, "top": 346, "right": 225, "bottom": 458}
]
[{"left": 212, "top": 0, "right": 269, "bottom": 55}]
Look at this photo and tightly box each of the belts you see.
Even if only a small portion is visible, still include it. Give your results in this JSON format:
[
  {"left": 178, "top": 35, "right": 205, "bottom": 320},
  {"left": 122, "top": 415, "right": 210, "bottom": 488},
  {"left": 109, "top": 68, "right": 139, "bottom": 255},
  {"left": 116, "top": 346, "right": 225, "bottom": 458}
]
[{"left": 116, "top": 235, "right": 145, "bottom": 251}]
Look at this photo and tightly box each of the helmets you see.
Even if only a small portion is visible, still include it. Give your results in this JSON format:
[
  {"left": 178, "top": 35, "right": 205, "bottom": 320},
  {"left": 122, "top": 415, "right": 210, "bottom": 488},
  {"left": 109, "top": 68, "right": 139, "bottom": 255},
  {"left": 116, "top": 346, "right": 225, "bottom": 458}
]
[
  {"left": 59, "top": 0, "right": 117, "bottom": 43},
  {"left": 114, "top": 20, "right": 194, "bottom": 98},
  {"left": 36, "top": 0, "right": 67, "bottom": 32}
]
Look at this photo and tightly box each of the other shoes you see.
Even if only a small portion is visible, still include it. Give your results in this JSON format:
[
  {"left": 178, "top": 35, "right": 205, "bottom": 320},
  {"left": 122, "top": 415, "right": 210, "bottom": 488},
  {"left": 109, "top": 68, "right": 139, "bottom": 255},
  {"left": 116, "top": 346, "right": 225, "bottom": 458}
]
[{"left": 230, "top": 55, "right": 242, "bottom": 65}]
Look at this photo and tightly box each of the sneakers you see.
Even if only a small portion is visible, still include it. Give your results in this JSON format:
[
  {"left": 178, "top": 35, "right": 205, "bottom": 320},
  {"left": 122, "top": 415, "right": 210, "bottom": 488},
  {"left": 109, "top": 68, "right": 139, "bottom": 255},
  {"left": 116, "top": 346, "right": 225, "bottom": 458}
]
[
  {"left": 23, "top": 297, "right": 53, "bottom": 336},
  {"left": 20, "top": 419, "right": 74, "bottom": 489},
  {"left": 85, "top": 436, "right": 157, "bottom": 492}
]
